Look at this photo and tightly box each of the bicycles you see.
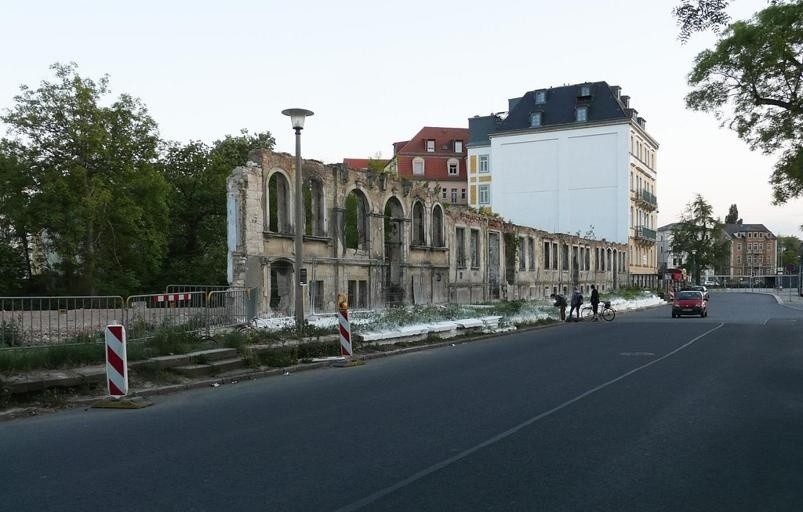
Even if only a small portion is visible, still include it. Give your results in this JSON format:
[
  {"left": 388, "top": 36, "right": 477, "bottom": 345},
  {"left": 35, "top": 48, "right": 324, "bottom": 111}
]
[{"left": 581, "top": 301, "right": 615, "bottom": 321}]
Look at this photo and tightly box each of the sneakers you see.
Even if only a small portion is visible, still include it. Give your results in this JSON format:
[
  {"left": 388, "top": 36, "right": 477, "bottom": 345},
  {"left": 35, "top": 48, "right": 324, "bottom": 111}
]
[
  {"left": 590, "top": 316, "right": 599, "bottom": 322},
  {"left": 559, "top": 318, "right": 565, "bottom": 322}
]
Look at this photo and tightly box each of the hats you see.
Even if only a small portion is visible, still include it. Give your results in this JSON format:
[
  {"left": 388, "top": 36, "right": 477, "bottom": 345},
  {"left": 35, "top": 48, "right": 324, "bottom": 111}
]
[{"left": 590, "top": 284, "right": 595, "bottom": 288}]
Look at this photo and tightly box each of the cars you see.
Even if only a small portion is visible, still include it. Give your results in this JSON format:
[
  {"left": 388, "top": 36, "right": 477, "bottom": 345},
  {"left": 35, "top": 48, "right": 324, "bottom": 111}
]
[{"left": 672, "top": 285, "right": 709, "bottom": 318}]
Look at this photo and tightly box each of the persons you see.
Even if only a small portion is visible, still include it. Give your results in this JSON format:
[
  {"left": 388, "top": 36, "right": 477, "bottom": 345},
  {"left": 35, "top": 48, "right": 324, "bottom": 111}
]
[
  {"left": 568, "top": 285, "right": 583, "bottom": 322},
  {"left": 590, "top": 284, "right": 600, "bottom": 324},
  {"left": 550, "top": 292, "right": 569, "bottom": 322}
]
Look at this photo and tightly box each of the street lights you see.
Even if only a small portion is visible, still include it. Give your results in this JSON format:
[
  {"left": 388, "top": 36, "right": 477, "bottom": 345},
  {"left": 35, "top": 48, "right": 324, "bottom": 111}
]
[{"left": 281, "top": 108, "right": 313, "bottom": 327}]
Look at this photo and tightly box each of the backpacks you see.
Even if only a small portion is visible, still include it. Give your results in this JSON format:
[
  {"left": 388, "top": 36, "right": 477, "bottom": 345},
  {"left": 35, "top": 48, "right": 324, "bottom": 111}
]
[
  {"left": 575, "top": 293, "right": 583, "bottom": 306},
  {"left": 560, "top": 297, "right": 567, "bottom": 307}
]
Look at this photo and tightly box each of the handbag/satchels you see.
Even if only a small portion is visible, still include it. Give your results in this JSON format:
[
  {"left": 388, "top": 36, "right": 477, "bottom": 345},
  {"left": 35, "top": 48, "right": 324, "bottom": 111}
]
[{"left": 591, "top": 295, "right": 599, "bottom": 304}]
